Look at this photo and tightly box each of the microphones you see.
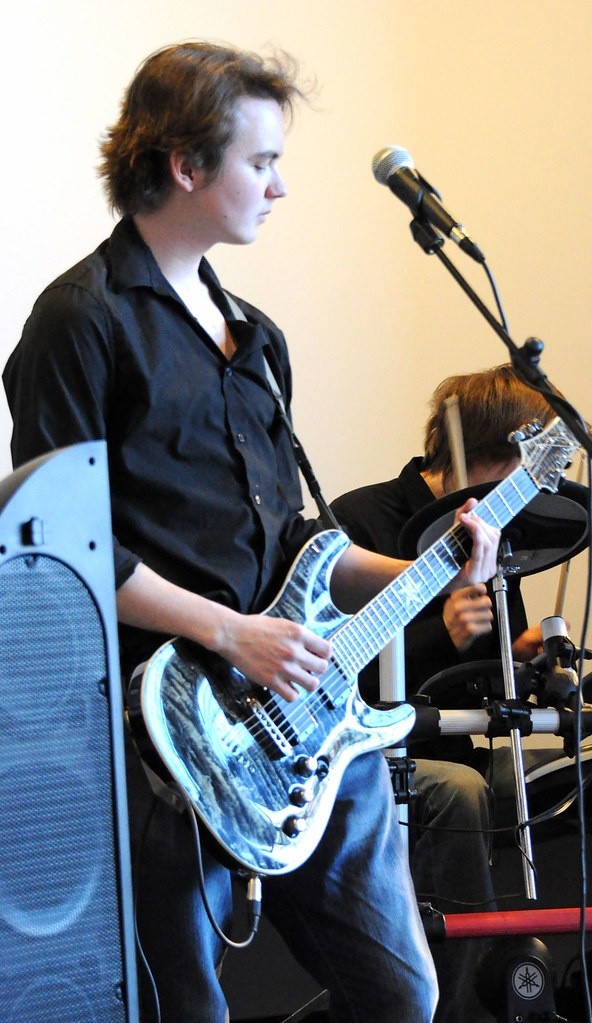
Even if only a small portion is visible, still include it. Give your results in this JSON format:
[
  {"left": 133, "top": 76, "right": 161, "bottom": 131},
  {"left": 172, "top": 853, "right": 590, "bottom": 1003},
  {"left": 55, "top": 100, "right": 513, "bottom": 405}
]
[
  {"left": 542, "top": 616, "right": 579, "bottom": 701},
  {"left": 372, "top": 144, "right": 486, "bottom": 263}
]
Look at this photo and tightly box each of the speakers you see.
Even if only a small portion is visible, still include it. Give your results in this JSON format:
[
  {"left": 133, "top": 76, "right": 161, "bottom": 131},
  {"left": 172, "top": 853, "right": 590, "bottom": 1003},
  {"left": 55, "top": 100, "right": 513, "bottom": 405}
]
[{"left": 0, "top": 440, "right": 141, "bottom": 1023}]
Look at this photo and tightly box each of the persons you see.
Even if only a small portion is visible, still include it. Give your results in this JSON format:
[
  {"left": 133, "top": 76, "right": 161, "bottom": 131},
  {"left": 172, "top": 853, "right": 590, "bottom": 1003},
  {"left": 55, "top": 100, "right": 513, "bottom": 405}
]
[
  {"left": 0, "top": 43, "right": 502, "bottom": 1023},
  {"left": 318, "top": 362, "right": 563, "bottom": 993}
]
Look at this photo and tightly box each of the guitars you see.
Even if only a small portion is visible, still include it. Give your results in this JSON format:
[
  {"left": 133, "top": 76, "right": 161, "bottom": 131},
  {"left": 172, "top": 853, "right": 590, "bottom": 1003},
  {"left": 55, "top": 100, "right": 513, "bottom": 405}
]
[{"left": 126, "top": 407, "right": 588, "bottom": 878}]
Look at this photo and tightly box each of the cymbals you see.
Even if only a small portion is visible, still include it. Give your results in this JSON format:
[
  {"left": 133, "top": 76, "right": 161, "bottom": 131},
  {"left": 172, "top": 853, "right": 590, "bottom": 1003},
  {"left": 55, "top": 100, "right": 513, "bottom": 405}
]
[
  {"left": 396, "top": 477, "right": 592, "bottom": 582},
  {"left": 416, "top": 659, "right": 541, "bottom": 710}
]
